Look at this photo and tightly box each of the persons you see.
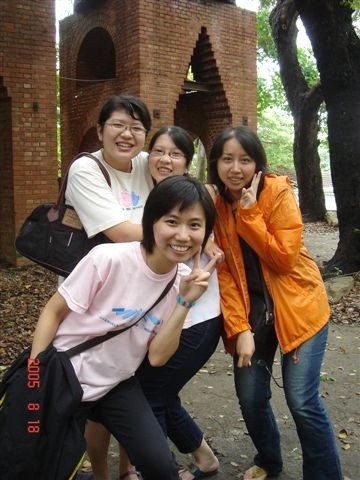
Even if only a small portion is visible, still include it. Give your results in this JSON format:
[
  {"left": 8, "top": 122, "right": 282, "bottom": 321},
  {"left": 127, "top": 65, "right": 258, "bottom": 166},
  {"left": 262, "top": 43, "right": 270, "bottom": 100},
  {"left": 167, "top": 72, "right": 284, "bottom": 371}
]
[
  {"left": 57, "top": 95, "right": 154, "bottom": 480},
  {"left": 26, "top": 172, "right": 222, "bottom": 480},
  {"left": 136, "top": 124, "right": 224, "bottom": 480},
  {"left": 206, "top": 126, "right": 342, "bottom": 480}
]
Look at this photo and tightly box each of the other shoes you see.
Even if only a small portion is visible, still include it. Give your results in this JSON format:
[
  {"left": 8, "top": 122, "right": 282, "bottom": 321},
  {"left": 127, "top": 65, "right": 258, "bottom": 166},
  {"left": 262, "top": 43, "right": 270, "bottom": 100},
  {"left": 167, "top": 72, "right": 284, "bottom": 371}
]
[{"left": 244, "top": 465, "right": 269, "bottom": 480}]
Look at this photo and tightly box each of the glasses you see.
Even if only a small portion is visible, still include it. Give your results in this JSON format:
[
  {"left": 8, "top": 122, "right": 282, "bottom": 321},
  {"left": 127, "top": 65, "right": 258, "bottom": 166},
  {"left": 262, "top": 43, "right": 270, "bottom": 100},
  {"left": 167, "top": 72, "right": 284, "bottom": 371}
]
[
  {"left": 149, "top": 149, "right": 187, "bottom": 159},
  {"left": 247, "top": 358, "right": 284, "bottom": 389},
  {"left": 104, "top": 122, "right": 148, "bottom": 135}
]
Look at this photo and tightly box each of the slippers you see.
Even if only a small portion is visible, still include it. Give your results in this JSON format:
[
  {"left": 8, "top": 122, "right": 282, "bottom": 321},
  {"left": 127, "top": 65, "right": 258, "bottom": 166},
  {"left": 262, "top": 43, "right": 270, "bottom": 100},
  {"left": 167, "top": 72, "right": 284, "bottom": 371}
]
[{"left": 178, "top": 462, "right": 219, "bottom": 480}]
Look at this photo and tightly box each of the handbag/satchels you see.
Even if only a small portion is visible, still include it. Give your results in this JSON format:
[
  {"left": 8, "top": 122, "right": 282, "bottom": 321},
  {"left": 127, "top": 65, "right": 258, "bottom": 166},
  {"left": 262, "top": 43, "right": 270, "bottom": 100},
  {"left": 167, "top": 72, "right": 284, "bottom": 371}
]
[
  {"left": 249, "top": 294, "right": 272, "bottom": 334},
  {"left": 15, "top": 152, "right": 114, "bottom": 277}
]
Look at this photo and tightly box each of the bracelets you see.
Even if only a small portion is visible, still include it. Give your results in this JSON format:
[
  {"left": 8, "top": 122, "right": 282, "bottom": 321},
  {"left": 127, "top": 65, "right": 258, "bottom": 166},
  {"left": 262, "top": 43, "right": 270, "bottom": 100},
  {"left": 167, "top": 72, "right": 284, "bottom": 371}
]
[{"left": 176, "top": 294, "right": 195, "bottom": 309}]
